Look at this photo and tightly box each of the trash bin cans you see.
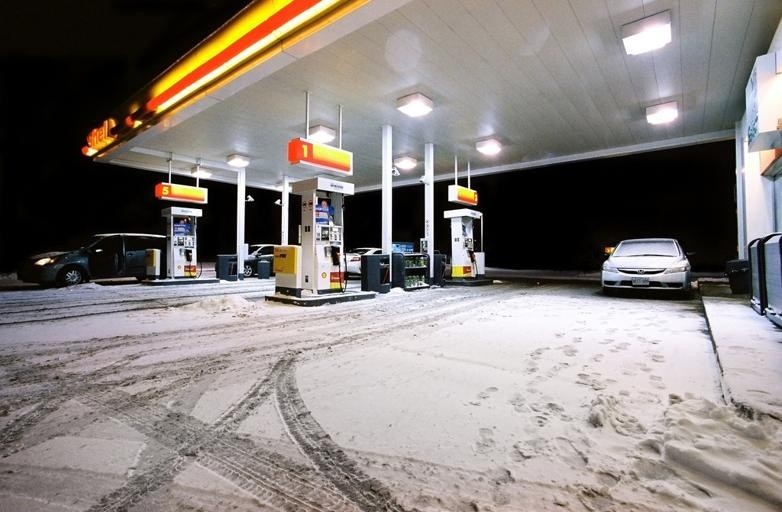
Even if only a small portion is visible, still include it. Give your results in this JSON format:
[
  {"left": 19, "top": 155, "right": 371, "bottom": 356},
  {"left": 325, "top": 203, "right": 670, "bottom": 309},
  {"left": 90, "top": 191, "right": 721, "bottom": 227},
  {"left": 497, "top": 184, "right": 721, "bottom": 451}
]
[
  {"left": 727, "top": 260, "right": 750, "bottom": 294},
  {"left": 257, "top": 261, "right": 271, "bottom": 279}
]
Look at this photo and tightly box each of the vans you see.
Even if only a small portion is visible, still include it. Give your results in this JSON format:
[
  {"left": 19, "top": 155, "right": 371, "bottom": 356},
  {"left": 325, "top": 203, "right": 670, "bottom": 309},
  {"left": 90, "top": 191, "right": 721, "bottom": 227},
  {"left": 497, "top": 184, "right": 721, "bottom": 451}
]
[{"left": 16, "top": 232, "right": 167, "bottom": 287}]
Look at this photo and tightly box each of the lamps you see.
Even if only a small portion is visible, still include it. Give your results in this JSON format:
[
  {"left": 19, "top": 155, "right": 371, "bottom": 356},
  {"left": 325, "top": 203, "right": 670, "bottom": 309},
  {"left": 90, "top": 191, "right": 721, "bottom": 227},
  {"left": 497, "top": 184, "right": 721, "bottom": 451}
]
[
  {"left": 308, "top": 125, "right": 335, "bottom": 143},
  {"left": 397, "top": 93, "right": 433, "bottom": 117},
  {"left": 394, "top": 157, "right": 417, "bottom": 169},
  {"left": 276, "top": 183, "right": 292, "bottom": 190},
  {"left": 475, "top": 139, "right": 501, "bottom": 156},
  {"left": 226, "top": 155, "right": 250, "bottom": 167},
  {"left": 646, "top": 102, "right": 678, "bottom": 123},
  {"left": 621, "top": 10, "right": 671, "bottom": 56}
]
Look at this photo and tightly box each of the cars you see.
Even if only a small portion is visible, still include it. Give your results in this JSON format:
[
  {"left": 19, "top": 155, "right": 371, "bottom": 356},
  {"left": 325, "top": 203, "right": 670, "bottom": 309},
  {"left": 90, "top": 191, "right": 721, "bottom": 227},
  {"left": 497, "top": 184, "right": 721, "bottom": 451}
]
[
  {"left": 230, "top": 244, "right": 279, "bottom": 277},
  {"left": 600, "top": 237, "right": 696, "bottom": 297},
  {"left": 345, "top": 247, "right": 382, "bottom": 280}
]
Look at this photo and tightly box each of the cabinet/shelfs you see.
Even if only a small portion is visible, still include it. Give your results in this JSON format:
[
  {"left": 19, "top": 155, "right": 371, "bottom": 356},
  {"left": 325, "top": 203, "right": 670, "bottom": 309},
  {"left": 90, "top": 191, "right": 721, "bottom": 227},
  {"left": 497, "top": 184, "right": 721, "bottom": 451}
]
[{"left": 393, "top": 252, "right": 430, "bottom": 291}]
